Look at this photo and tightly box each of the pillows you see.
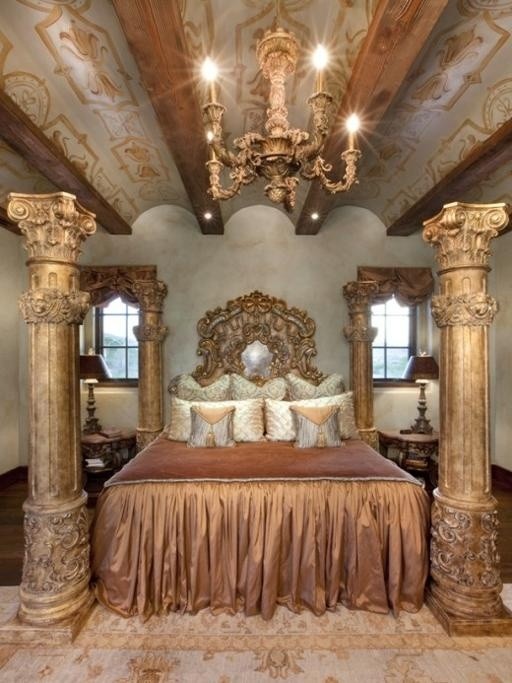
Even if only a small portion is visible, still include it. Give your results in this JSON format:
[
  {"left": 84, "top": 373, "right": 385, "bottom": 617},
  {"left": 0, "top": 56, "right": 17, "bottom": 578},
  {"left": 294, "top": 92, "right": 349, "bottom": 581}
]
[{"left": 169, "top": 374, "right": 359, "bottom": 447}]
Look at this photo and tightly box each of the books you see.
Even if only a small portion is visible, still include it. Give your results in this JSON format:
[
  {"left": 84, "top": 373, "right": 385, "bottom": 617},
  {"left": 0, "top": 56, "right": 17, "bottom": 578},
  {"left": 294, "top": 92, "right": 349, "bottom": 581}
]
[
  {"left": 99, "top": 426, "right": 123, "bottom": 438},
  {"left": 404, "top": 457, "right": 428, "bottom": 468},
  {"left": 85, "top": 457, "right": 111, "bottom": 469}
]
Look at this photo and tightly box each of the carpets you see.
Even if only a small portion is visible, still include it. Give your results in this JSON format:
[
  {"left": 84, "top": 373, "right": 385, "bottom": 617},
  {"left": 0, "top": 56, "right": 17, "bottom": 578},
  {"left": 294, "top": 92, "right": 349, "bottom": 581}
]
[{"left": 0, "top": 583, "right": 512, "bottom": 683}]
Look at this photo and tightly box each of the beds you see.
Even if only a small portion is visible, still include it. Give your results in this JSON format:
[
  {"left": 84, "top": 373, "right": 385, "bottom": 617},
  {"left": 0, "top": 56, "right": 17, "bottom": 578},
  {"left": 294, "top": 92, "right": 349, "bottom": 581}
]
[{"left": 94, "top": 289, "right": 432, "bottom": 617}]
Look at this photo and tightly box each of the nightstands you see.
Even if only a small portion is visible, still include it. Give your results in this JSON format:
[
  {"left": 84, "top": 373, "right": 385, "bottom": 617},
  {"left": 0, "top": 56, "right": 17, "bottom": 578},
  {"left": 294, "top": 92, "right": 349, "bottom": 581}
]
[
  {"left": 377, "top": 429, "right": 439, "bottom": 488},
  {"left": 81, "top": 427, "right": 137, "bottom": 489}
]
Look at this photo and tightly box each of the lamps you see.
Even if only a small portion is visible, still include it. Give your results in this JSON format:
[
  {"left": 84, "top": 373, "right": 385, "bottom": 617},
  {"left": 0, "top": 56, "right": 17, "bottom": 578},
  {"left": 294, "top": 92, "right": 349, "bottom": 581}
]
[
  {"left": 74, "top": 351, "right": 111, "bottom": 439},
  {"left": 400, "top": 354, "right": 438, "bottom": 432},
  {"left": 188, "top": 31, "right": 364, "bottom": 217}
]
